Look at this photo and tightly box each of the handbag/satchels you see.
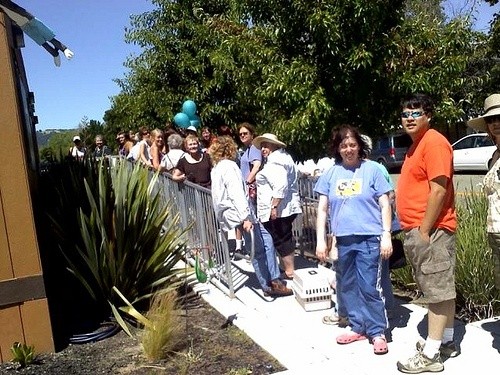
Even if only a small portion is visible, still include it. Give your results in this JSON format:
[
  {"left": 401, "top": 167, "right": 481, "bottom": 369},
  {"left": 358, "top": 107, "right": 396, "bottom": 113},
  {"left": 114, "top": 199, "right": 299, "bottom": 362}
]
[{"left": 247, "top": 179, "right": 257, "bottom": 210}]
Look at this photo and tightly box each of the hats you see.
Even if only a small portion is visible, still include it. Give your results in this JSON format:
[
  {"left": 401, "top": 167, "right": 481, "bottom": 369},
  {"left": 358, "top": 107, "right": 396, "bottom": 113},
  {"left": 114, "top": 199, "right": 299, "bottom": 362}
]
[
  {"left": 468, "top": 94, "right": 500, "bottom": 132},
  {"left": 252, "top": 133, "right": 287, "bottom": 149},
  {"left": 73, "top": 135, "right": 81, "bottom": 142},
  {"left": 185, "top": 126, "right": 197, "bottom": 133}
]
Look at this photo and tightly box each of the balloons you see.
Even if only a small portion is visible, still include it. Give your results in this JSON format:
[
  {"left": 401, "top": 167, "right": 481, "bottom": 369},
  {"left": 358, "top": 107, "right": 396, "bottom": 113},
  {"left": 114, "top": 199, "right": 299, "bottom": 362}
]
[{"left": 173, "top": 101, "right": 201, "bottom": 129}]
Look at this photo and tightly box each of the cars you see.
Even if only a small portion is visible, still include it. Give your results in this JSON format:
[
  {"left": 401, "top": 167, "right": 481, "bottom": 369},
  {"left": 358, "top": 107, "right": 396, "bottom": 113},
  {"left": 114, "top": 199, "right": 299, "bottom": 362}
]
[{"left": 450, "top": 132, "right": 498, "bottom": 172}]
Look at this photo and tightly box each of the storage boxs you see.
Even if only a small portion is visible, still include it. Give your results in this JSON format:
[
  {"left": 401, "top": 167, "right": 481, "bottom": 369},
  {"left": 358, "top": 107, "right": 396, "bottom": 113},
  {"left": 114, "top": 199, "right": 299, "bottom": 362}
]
[{"left": 292, "top": 268, "right": 333, "bottom": 311}]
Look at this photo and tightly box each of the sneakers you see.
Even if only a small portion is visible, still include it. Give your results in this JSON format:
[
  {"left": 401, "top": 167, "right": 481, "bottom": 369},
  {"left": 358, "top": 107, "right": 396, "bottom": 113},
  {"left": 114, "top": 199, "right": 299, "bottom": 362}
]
[
  {"left": 396, "top": 352, "right": 444, "bottom": 374},
  {"left": 415, "top": 339, "right": 460, "bottom": 357}
]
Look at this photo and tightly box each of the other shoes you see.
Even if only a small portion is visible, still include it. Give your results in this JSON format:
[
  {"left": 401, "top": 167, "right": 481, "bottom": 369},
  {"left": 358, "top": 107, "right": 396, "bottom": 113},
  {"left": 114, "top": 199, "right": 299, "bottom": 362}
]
[
  {"left": 264, "top": 280, "right": 293, "bottom": 297},
  {"left": 371, "top": 335, "right": 389, "bottom": 354},
  {"left": 323, "top": 313, "right": 349, "bottom": 326},
  {"left": 336, "top": 331, "right": 368, "bottom": 344},
  {"left": 279, "top": 266, "right": 294, "bottom": 279},
  {"left": 233, "top": 249, "right": 250, "bottom": 261}
]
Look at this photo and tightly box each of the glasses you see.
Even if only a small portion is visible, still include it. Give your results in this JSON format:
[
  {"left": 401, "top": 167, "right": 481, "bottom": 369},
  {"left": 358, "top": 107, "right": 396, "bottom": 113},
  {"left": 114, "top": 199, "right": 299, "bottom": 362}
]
[
  {"left": 143, "top": 132, "right": 149, "bottom": 135},
  {"left": 239, "top": 132, "right": 248, "bottom": 136},
  {"left": 484, "top": 115, "right": 500, "bottom": 123},
  {"left": 401, "top": 112, "right": 424, "bottom": 118}
]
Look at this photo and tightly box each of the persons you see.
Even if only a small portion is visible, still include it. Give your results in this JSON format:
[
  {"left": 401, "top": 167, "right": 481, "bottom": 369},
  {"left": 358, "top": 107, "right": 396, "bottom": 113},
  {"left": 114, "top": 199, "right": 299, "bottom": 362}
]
[
  {"left": 68, "top": 123, "right": 395, "bottom": 354},
  {"left": 397, "top": 94, "right": 459, "bottom": 373},
  {"left": 466, "top": 95, "right": 500, "bottom": 291}
]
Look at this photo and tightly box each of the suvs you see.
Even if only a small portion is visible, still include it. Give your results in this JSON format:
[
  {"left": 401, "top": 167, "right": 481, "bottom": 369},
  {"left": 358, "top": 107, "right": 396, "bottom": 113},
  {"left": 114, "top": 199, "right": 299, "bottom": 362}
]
[{"left": 369, "top": 132, "right": 414, "bottom": 169}]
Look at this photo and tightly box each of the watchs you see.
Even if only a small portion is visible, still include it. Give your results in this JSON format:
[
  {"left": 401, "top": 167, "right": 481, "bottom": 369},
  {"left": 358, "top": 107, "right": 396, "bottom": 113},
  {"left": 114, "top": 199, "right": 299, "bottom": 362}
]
[
  {"left": 382, "top": 228, "right": 392, "bottom": 233},
  {"left": 271, "top": 205, "right": 277, "bottom": 209}
]
